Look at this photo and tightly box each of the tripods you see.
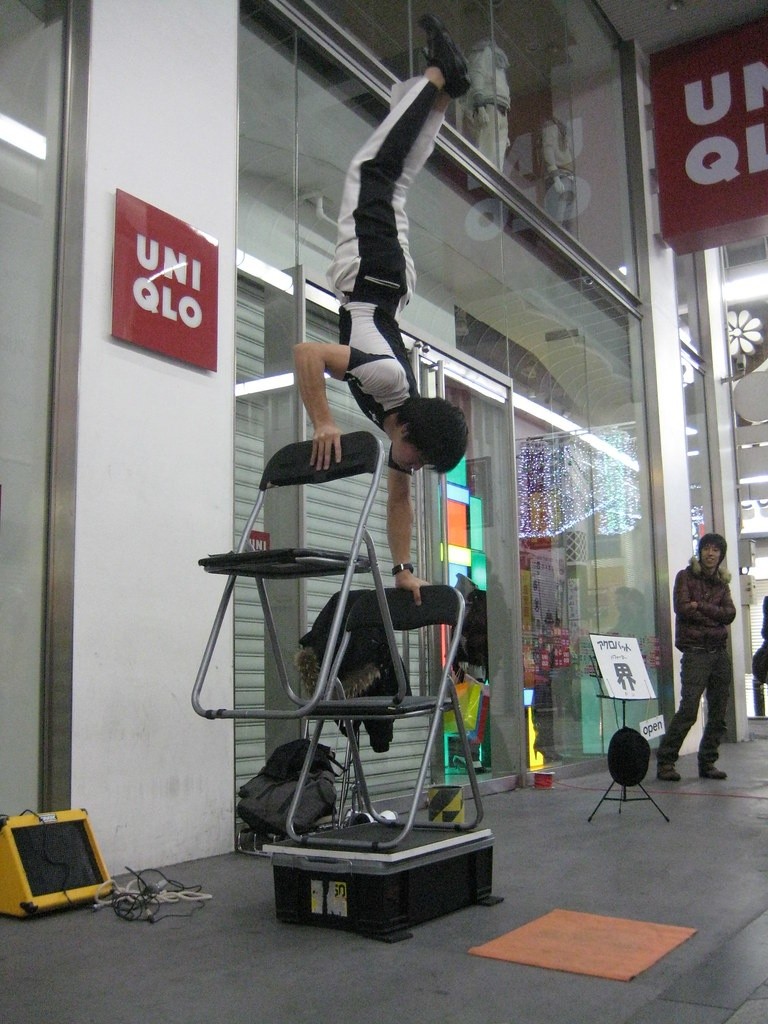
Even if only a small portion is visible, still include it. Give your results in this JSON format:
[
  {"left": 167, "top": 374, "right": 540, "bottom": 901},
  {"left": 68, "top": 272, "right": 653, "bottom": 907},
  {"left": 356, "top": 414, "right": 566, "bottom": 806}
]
[{"left": 587, "top": 631, "right": 669, "bottom": 824}]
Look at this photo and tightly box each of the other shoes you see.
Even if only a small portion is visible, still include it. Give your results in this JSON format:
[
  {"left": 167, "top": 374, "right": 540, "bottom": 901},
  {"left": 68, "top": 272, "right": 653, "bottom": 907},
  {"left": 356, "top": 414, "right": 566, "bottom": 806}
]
[
  {"left": 698, "top": 764, "right": 727, "bottom": 780},
  {"left": 657, "top": 765, "right": 681, "bottom": 782},
  {"left": 421, "top": 13, "right": 472, "bottom": 99}
]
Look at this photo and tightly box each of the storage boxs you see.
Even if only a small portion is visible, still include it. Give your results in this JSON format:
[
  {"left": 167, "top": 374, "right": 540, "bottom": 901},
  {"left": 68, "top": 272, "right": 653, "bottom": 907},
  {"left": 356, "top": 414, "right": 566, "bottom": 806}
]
[{"left": 272, "top": 835, "right": 505, "bottom": 944}]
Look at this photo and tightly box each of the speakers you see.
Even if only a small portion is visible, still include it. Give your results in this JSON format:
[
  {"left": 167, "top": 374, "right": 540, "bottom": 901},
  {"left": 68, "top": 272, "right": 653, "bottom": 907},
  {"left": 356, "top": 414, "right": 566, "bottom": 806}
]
[{"left": 0, "top": 807, "right": 115, "bottom": 916}]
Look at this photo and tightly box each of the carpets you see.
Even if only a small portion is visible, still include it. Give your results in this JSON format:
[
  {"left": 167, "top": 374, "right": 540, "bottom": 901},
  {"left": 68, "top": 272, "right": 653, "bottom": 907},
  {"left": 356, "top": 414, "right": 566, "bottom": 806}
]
[{"left": 467, "top": 907, "right": 700, "bottom": 983}]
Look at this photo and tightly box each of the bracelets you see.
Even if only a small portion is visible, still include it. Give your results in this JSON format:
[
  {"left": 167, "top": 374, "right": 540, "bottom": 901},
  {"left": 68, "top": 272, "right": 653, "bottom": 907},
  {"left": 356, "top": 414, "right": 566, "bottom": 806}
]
[{"left": 392, "top": 563, "right": 414, "bottom": 576}]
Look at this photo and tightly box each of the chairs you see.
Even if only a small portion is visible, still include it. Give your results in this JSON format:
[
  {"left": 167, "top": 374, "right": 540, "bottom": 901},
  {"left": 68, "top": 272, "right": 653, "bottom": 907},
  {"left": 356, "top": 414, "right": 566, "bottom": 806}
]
[
  {"left": 189, "top": 430, "right": 408, "bottom": 720},
  {"left": 283, "top": 585, "right": 486, "bottom": 851}
]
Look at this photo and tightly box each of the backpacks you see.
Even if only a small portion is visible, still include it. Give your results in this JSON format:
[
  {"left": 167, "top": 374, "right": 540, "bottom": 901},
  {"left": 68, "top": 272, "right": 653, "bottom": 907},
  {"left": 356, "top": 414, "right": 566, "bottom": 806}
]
[{"left": 237, "top": 742, "right": 338, "bottom": 835}]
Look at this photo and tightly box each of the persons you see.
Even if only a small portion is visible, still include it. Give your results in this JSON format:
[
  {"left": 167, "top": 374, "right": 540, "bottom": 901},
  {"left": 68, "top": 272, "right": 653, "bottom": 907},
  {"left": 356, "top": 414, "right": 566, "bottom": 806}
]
[
  {"left": 296, "top": 14, "right": 472, "bottom": 609},
  {"left": 468, "top": 36, "right": 510, "bottom": 172},
  {"left": 616, "top": 587, "right": 646, "bottom": 636},
  {"left": 656, "top": 533, "right": 737, "bottom": 780},
  {"left": 762, "top": 596, "right": 768, "bottom": 643},
  {"left": 542, "top": 119, "right": 573, "bottom": 233}
]
